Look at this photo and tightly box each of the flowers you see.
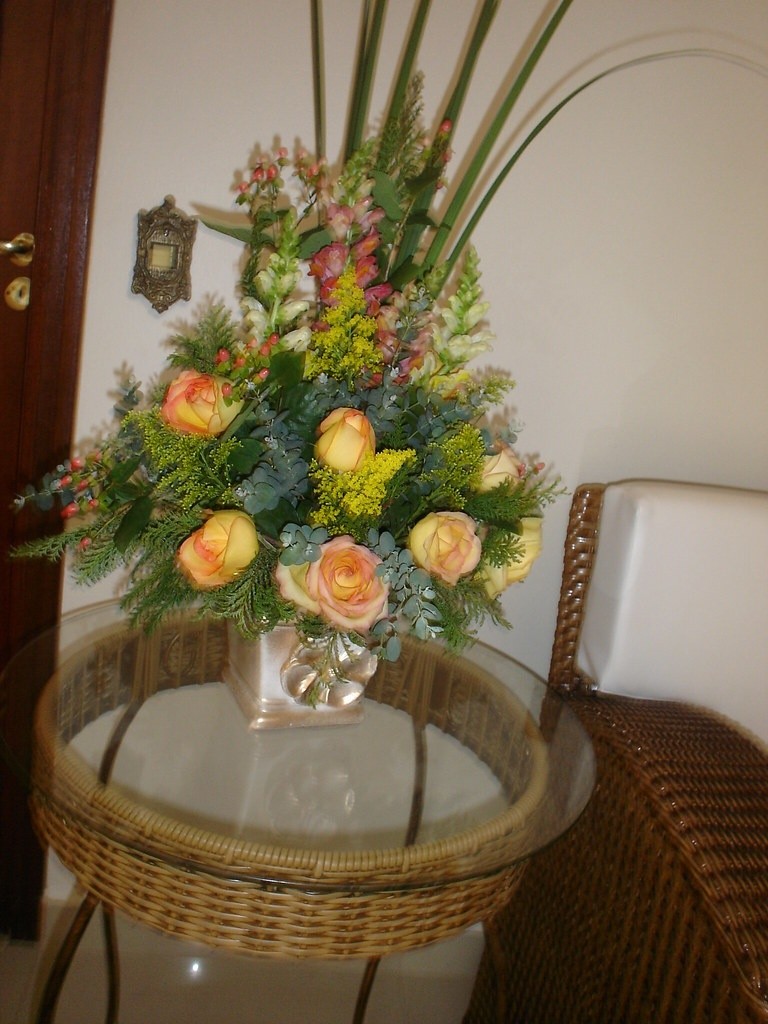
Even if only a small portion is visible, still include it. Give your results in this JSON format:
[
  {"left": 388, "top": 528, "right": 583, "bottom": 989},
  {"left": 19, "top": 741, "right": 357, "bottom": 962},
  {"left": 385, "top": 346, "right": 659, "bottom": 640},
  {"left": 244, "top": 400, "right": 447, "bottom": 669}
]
[{"left": 11, "top": 2, "right": 698, "bottom": 655}]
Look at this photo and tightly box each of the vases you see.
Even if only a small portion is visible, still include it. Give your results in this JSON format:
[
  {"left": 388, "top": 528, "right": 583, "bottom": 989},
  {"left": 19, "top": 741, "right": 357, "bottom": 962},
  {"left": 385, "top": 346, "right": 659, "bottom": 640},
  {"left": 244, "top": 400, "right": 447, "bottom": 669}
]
[{"left": 221, "top": 602, "right": 365, "bottom": 730}]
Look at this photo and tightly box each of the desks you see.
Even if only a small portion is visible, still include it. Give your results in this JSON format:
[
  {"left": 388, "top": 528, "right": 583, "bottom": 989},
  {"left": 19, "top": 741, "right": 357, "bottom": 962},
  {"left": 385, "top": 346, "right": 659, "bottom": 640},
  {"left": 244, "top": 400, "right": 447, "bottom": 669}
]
[{"left": 0, "top": 595, "right": 601, "bottom": 1024}]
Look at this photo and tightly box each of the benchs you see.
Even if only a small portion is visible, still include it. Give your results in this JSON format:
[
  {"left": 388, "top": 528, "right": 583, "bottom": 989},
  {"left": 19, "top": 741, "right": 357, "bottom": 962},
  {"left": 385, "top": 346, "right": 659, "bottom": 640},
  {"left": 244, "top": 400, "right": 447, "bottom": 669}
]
[{"left": 460, "top": 482, "right": 768, "bottom": 1024}]
